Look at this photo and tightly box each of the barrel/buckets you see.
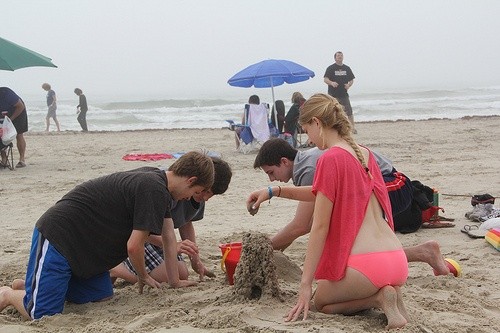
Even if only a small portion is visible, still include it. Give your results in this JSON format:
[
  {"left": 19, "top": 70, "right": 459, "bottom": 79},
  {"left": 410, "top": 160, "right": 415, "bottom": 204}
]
[{"left": 219, "top": 242, "right": 242, "bottom": 286}]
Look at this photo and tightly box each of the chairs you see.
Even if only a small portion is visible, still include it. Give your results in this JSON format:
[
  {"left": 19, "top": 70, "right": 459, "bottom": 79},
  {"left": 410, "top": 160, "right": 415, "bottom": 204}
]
[
  {"left": 0, "top": 111, "right": 13, "bottom": 171},
  {"left": 294, "top": 98, "right": 316, "bottom": 148},
  {"left": 226, "top": 104, "right": 269, "bottom": 154}
]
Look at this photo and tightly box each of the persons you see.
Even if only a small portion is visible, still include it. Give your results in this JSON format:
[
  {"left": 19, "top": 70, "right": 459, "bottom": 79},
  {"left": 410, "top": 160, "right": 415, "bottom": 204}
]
[
  {"left": 1, "top": 87, "right": 28, "bottom": 168},
  {"left": 247, "top": 93, "right": 408, "bottom": 329},
  {"left": 253, "top": 139, "right": 450, "bottom": 276},
  {"left": 228, "top": 91, "right": 308, "bottom": 152},
  {"left": 42, "top": 83, "right": 60, "bottom": 132},
  {"left": 74, "top": 88, "right": 89, "bottom": 132},
  {"left": 324, "top": 51, "right": 358, "bottom": 135},
  {"left": 108, "top": 155, "right": 232, "bottom": 282},
  {"left": 1, "top": 150, "right": 215, "bottom": 319}
]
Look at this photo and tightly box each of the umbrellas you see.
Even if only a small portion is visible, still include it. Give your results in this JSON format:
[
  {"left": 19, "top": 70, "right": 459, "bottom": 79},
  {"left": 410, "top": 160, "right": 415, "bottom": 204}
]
[
  {"left": 1, "top": 36, "right": 58, "bottom": 71},
  {"left": 228, "top": 59, "right": 315, "bottom": 130}
]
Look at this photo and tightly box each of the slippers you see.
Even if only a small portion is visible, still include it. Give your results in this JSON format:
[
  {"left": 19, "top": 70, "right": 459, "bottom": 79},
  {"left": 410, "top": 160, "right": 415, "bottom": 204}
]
[
  {"left": 16, "top": 162, "right": 26, "bottom": 168},
  {"left": 422, "top": 215, "right": 456, "bottom": 228},
  {"left": 461, "top": 224, "right": 485, "bottom": 239}
]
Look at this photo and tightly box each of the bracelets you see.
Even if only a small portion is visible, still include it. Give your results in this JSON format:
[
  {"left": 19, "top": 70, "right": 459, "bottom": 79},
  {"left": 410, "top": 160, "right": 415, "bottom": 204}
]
[
  {"left": 278, "top": 185, "right": 281, "bottom": 197},
  {"left": 268, "top": 186, "right": 272, "bottom": 203}
]
